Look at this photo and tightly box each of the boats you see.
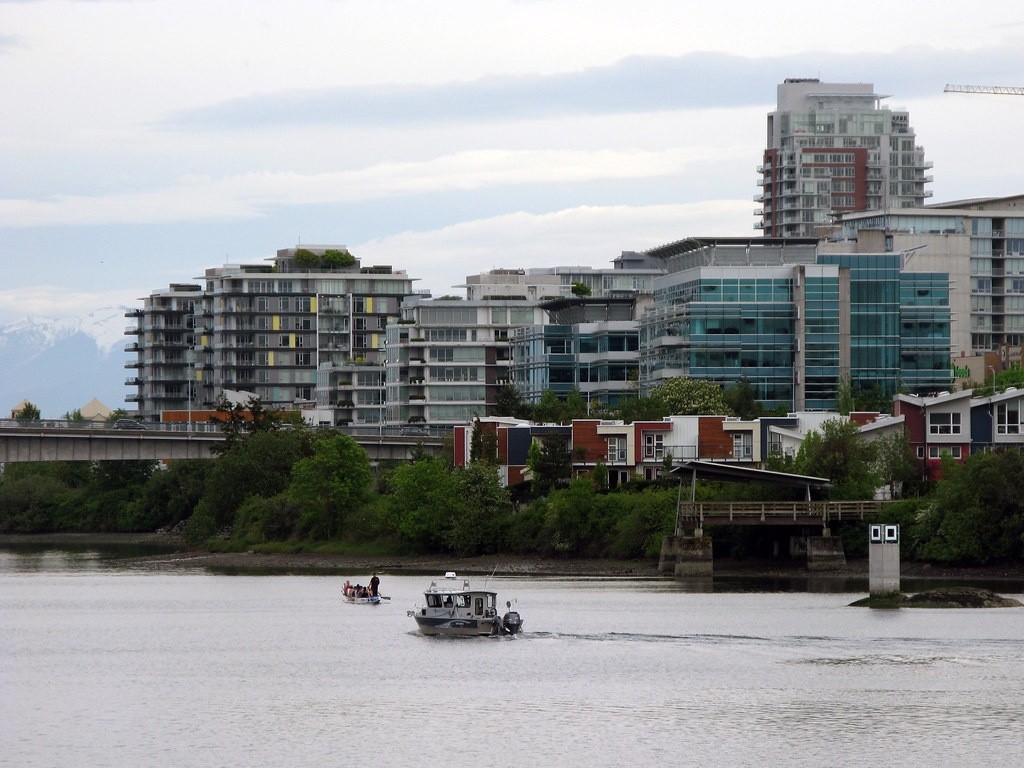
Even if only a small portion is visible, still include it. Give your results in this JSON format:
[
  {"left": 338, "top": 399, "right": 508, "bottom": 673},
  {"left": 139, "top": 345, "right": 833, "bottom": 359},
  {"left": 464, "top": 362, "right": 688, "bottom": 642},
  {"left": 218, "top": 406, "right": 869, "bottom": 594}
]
[
  {"left": 341, "top": 580, "right": 382, "bottom": 604},
  {"left": 405, "top": 577, "right": 524, "bottom": 638}
]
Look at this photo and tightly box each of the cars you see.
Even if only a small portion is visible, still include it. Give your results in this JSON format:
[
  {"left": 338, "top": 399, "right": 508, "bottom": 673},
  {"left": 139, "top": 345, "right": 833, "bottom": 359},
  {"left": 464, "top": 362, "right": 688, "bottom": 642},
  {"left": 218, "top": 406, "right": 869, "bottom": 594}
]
[
  {"left": 111, "top": 419, "right": 148, "bottom": 430},
  {"left": 399, "top": 426, "right": 430, "bottom": 436}
]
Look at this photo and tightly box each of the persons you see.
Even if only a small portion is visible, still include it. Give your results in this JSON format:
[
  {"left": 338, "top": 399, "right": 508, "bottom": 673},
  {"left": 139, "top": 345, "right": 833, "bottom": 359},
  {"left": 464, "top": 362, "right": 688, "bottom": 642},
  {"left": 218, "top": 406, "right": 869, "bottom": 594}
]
[
  {"left": 446, "top": 594, "right": 453, "bottom": 607},
  {"left": 341, "top": 572, "right": 380, "bottom": 597}
]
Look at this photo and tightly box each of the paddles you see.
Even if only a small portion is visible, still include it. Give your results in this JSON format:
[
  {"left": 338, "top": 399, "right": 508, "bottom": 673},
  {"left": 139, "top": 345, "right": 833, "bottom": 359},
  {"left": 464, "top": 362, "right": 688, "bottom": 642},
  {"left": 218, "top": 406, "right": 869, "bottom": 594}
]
[{"left": 381, "top": 597, "right": 392, "bottom": 600}]
